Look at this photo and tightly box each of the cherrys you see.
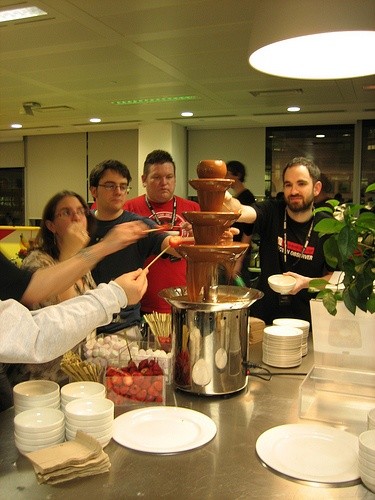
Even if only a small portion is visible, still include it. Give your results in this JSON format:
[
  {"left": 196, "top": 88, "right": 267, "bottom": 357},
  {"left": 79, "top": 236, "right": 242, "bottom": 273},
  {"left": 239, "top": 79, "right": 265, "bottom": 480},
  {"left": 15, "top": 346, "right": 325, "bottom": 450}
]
[
  {"left": 173, "top": 351, "right": 192, "bottom": 386},
  {"left": 169, "top": 236, "right": 180, "bottom": 248},
  {"left": 105, "top": 358, "right": 165, "bottom": 405}
]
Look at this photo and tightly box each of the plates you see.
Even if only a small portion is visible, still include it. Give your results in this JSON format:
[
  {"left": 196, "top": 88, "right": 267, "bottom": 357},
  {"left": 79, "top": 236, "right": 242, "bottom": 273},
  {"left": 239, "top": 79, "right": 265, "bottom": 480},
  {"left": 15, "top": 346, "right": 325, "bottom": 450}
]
[
  {"left": 255, "top": 424, "right": 361, "bottom": 483},
  {"left": 261, "top": 318, "right": 310, "bottom": 368},
  {"left": 110, "top": 406, "right": 217, "bottom": 454}
]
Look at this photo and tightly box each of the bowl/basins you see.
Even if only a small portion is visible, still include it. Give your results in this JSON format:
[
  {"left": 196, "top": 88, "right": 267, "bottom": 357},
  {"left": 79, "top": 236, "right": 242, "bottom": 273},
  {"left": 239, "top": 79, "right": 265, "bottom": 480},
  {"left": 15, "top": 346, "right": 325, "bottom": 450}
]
[
  {"left": 267, "top": 274, "right": 296, "bottom": 294},
  {"left": 12, "top": 379, "right": 114, "bottom": 459},
  {"left": 359, "top": 408, "right": 375, "bottom": 493}
]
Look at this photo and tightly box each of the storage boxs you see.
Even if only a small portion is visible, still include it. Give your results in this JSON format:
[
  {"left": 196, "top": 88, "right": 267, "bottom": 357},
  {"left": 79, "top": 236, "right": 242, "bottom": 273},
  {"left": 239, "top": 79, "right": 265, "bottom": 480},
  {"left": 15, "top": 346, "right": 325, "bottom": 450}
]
[{"left": 79, "top": 334, "right": 174, "bottom": 407}]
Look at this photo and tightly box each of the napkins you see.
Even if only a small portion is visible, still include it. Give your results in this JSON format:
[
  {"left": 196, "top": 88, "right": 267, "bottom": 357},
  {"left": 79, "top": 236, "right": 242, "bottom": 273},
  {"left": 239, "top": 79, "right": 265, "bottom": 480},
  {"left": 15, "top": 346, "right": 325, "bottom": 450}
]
[
  {"left": 250, "top": 317, "right": 265, "bottom": 343},
  {"left": 29, "top": 431, "right": 111, "bottom": 485}
]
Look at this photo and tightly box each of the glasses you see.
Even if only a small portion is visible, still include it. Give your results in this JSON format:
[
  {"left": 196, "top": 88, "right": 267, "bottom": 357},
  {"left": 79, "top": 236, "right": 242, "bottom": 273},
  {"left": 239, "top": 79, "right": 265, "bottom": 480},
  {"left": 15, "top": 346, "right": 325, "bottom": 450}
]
[
  {"left": 54, "top": 207, "right": 87, "bottom": 217},
  {"left": 98, "top": 182, "right": 129, "bottom": 191}
]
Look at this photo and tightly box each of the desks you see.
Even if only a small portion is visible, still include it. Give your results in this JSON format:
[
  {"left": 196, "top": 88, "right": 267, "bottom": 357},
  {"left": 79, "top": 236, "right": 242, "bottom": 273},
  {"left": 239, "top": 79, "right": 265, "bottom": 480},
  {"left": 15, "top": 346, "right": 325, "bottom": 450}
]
[{"left": 0, "top": 326, "right": 375, "bottom": 500}]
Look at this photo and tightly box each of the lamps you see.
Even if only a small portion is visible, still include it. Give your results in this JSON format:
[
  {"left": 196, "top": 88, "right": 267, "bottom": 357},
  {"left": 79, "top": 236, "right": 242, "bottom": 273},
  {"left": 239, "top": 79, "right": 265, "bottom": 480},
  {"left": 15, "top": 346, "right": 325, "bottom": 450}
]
[{"left": 249, "top": 0, "right": 375, "bottom": 79}]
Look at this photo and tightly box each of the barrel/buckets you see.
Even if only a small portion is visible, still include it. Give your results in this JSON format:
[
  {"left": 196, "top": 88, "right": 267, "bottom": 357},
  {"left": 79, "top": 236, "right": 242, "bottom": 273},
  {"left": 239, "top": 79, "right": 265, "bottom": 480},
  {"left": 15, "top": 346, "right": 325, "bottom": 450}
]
[{"left": 171, "top": 306, "right": 250, "bottom": 396}]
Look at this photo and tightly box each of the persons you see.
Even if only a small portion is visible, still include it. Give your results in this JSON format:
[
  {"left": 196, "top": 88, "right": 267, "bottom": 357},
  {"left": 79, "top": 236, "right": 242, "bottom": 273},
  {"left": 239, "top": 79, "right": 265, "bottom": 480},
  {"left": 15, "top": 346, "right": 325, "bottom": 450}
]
[
  {"left": 120, "top": 150, "right": 240, "bottom": 338},
  {"left": 19, "top": 191, "right": 97, "bottom": 383},
  {"left": 0, "top": 219, "right": 149, "bottom": 410},
  {"left": 84, "top": 159, "right": 183, "bottom": 337},
  {"left": 221, "top": 157, "right": 368, "bottom": 332}
]
